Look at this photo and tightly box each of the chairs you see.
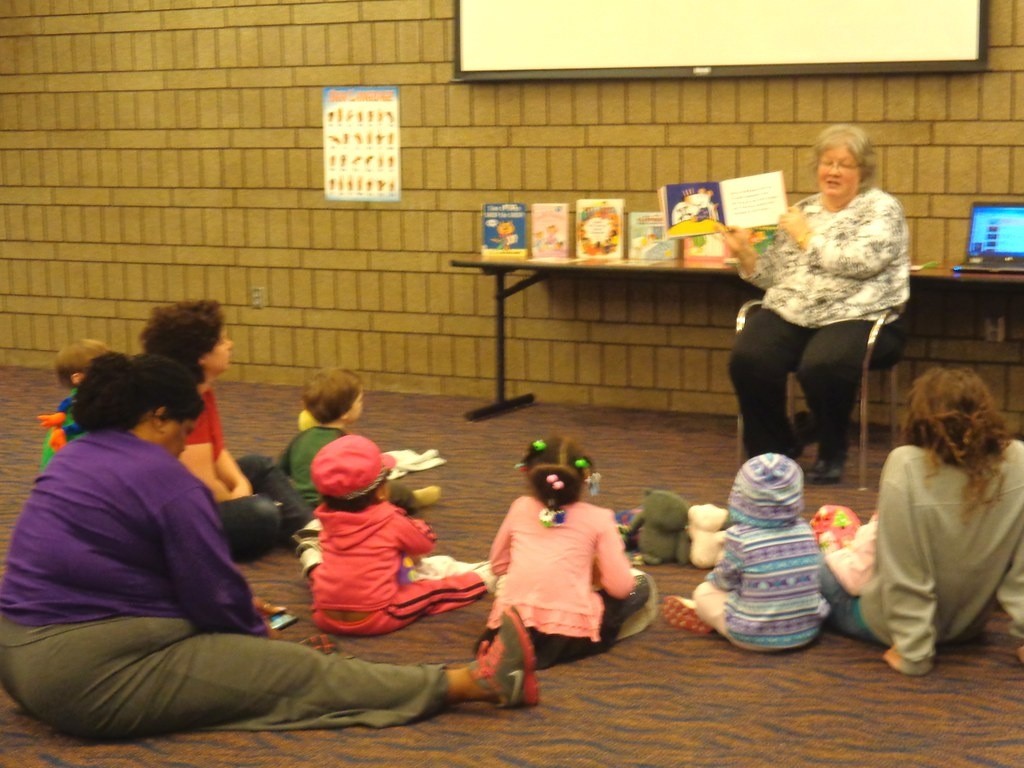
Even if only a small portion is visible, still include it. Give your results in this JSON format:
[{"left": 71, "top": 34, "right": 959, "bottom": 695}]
[{"left": 735, "top": 299, "right": 897, "bottom": 491}]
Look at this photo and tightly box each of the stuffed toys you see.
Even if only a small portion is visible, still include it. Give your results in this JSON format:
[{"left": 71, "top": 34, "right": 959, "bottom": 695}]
[
  {"left": 627, "top": 490, "right": 731, "bottom": 569},
  {"left": 40, "top": 394, "right": 82, "bottom": 450}
]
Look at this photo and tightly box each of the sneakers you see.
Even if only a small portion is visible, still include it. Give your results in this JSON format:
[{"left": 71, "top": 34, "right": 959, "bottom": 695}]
[
  {"left": 297, "top": 636, "right": 340, "bottom": 659},
  {"left": 472, "top": 604, "right": 538, "bottom": 707}
]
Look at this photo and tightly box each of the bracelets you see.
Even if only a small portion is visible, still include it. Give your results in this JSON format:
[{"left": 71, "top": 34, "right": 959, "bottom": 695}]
[{"left": 797, "top": 230, "right": 810, "bottom": 246}]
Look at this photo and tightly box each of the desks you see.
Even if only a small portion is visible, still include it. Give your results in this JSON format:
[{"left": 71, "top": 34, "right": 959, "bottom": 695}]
[{"left": 445, "top": 258, "right": 1024, "bottom": 421}]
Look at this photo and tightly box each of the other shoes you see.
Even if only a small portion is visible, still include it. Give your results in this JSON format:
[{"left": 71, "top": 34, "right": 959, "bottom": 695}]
[
  {"left": 661, "top": 595, "right": 713, "bottom": 634},
  {"left": 807, "top": 444, "right": 848, "bottom": 483},
  {"left": 476, "top": 564, "right": 498, "bottom": 592},
  {"left": 299, "top": 551, "right": 321, "bottom": 578}
]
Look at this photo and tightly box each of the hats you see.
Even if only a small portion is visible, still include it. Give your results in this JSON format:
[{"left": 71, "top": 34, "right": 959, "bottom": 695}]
[
  {"left": 311, "top": 435, "right": 396, "bottom": 498},
  {"left": 728, "top": 453, "right": 805, "bottom": 528}
]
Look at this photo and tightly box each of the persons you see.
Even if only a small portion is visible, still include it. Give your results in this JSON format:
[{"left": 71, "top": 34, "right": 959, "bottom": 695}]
[
  {"left": 480, "top": 437, "right": 655, "bottom": 669},
  {"left": 294, "top": 433, "right": 498, "bottom": 634},
  {"left": 720, "top": 124, "right": 911, "bottom": 485},
  {"left": 281, "top": 368, "right": 440, "bottom": 514},
  {"left": 662, "top": 453, "right": 828, "bottom": 652},
  {"left": 39, "top": 341, "right": 115, "bottom": 471},
  {"left": 138, "top": 299, "right": 314, "bottom": 564},
  {"left": 821, "top": 367, "right": 1024, "bottom": 675},
  {"left": 0, "top": 353, "right": 539, "bottom": 738}
]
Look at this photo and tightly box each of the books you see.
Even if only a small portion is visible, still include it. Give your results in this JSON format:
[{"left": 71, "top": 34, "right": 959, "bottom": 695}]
[{"left": 481, "top": 170, "right": 788, "bottom": 260}]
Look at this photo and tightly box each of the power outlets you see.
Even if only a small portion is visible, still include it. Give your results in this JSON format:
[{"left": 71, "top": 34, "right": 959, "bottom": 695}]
[{"left": 984, "top": 315, "right": 1005, "bottom": 344}]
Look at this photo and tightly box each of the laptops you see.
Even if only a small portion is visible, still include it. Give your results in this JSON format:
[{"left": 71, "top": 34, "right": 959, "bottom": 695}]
[{"left": 953, "top": 202, "right": 1024, "bottom": 274}]
